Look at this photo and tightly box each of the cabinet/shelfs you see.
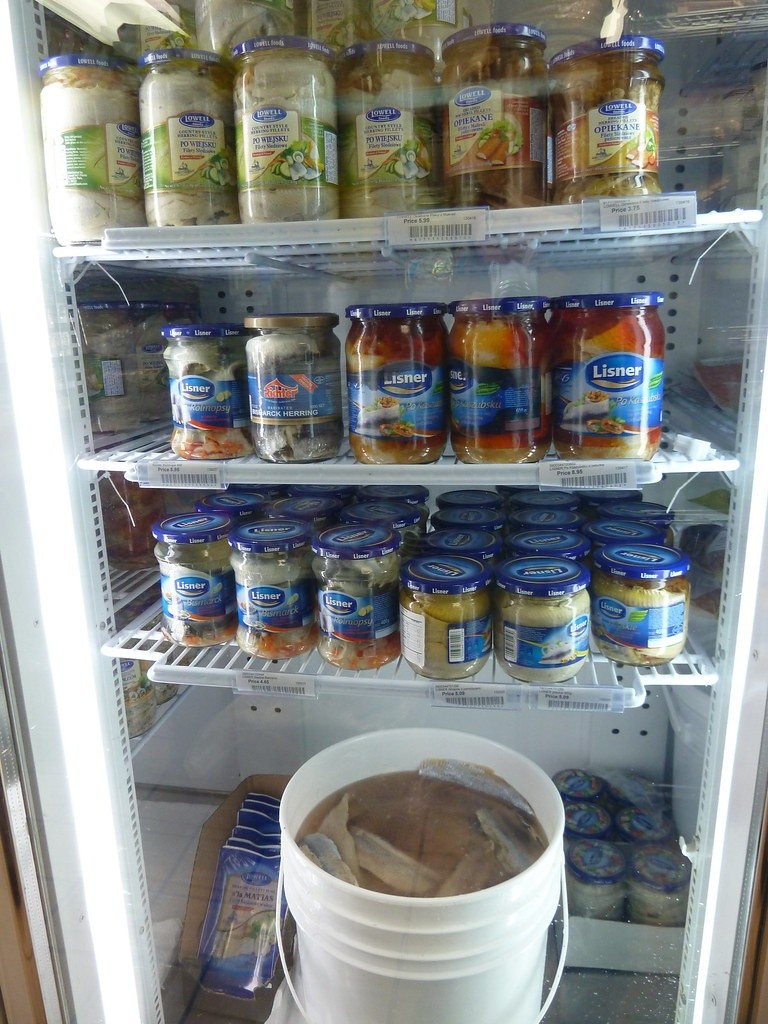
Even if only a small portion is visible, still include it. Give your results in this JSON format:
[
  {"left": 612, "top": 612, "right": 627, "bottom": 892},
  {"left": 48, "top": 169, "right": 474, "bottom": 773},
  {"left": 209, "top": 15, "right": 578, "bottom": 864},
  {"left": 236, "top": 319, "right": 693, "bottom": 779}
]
[{"left": 0, "top": 1, "right": 768, "bottom": 1024}]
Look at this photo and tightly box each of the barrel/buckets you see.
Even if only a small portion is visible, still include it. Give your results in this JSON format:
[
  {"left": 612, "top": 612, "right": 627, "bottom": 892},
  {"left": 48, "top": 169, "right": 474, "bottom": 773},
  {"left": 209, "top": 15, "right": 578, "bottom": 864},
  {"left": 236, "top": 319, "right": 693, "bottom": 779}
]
[{"left": 275, "top": 728, "right": 569, "bottom": 1024}]
